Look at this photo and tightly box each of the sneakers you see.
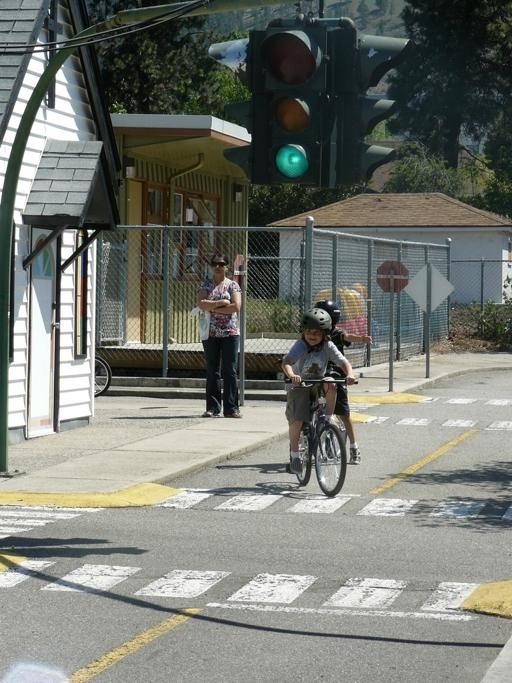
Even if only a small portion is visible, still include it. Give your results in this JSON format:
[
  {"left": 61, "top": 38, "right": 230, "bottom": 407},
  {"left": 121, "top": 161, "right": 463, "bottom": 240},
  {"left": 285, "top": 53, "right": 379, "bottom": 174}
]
[
  {"left": 224, "top": 411, "right": 240, "bottom": 417},
  {"left": 205, "top": 410, "right": 214, "bottom": 417},
  {"left": 350, "top": 446, "right": 361, "bottom": 464},
  {"left": 290, "top": 455, "right": 302, "bottom": 473}
]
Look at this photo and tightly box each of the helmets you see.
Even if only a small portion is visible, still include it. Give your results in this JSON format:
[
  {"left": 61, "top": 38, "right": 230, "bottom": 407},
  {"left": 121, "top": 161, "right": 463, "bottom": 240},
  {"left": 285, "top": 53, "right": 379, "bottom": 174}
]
[
  {"left": 315, "top": 299, "right": 339, "bottom": 322},
  {"left": 298, "top": 308, "right": 332, "bottom": 333}
]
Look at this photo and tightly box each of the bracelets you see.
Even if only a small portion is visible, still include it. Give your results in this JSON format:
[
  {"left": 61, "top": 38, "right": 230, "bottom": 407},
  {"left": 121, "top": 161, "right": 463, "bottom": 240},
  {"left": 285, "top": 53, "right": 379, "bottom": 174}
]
[{"left": 214, "top": 308, "right": 216, "bottom": 312}]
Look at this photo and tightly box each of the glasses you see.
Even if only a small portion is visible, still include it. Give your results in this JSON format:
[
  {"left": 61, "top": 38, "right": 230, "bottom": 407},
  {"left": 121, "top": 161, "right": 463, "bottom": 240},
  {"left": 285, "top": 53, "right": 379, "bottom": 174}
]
[{"left": 211, "top": 261, "right": 225, "bottom": 267}]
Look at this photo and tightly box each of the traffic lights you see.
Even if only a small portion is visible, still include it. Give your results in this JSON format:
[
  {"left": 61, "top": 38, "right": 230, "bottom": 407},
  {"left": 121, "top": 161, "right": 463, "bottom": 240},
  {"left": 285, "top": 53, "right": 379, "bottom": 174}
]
[
  {"left": 249, "top": 24, "right": 326, "bottom": 187},
  {"left": 335, "top": 29, "right": 411, "bottom": 189}
]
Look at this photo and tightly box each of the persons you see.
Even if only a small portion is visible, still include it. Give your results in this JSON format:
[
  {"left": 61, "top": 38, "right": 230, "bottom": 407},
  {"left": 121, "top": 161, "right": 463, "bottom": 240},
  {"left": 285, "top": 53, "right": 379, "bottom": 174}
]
[
  {"left": 313, "top": 299, "right": 372, "bottom": 466},
  {"left": 281, "top": 307, "right": 355, "bottom": 477},
  {"left": 198, "top": 252, "right": 243, "bottom": 418}
]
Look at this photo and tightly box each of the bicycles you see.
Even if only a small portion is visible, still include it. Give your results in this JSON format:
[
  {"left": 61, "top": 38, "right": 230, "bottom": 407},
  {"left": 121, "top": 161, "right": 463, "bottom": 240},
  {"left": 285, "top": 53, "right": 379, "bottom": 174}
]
[
  {"left": 95, "top": 352, "right": 112, "bottom": 397},
  {"left": 282, "top": 377, "right": 360, "bottom": 500}
]
